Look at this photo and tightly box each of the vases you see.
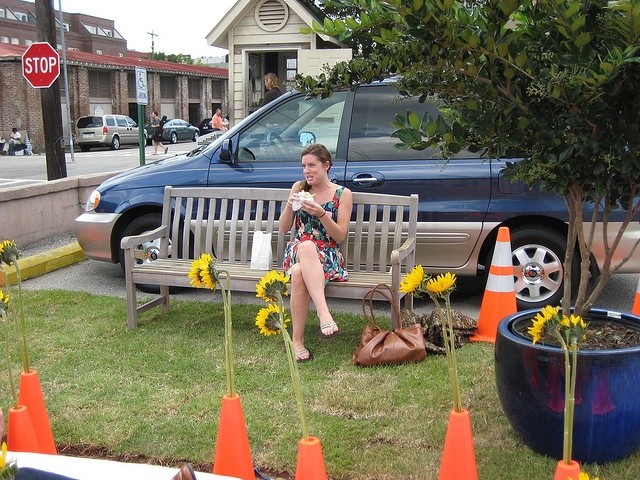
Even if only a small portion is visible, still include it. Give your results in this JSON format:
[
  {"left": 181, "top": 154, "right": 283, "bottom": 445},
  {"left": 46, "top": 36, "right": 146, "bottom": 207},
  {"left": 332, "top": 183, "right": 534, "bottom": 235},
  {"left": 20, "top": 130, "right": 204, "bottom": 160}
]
[
  {"left": 438, "top": 409, "right": 478, "bottom": 480},
  {"left": 213, "top": 393, "right": 255, "bottom": 480},
  {"left": 554, "top": 460, "right": 580, "bottom": 480},
  {"left": 295, "top": 436, "right": 326, "bottom": 480},
  {"left": 19, "top": 370, "right": 58, "bottom": 455},
  {"left": 7, "top": 405, "right": 40, "bottom": 453}
]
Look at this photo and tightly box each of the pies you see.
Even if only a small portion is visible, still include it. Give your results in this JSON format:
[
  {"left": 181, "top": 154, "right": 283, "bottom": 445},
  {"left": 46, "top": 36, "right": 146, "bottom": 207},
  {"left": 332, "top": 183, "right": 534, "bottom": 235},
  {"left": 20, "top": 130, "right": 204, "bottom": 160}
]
[{"left": 295, "top": 190, "right": 316, "bottom": 204}]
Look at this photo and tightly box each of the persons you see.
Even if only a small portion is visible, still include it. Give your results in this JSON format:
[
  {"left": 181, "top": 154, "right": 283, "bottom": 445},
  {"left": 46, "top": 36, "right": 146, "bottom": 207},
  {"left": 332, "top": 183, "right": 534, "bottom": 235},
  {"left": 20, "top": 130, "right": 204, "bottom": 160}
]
[
  {"left": 150, "top": 110, "right": 168, "bottom": 156},
  {"left": 210, "top": 108, "right": 229, "bottom": 132},
  {"left": 279, "top": 143, "right": 353, "bottom": 363},
  {"left": 0, "top": 128, "right": 21, "bottom": 155},
  {"left": 258, "top": 74, "right": 283, "bottom": 112}
]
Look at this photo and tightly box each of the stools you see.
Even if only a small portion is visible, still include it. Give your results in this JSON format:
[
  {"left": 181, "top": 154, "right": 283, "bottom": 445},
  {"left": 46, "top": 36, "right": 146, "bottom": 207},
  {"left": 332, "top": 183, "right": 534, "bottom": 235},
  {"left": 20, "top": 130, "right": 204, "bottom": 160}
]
[{"left": 8, "top": 143, "right": 24, "bottom": 156}]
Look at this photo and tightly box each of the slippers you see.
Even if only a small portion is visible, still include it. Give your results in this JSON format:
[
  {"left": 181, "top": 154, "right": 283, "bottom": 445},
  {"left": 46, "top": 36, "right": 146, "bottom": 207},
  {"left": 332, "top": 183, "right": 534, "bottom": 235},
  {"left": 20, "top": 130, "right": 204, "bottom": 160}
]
[
  {"left": 318, "top": 321, "right": 340, "bottom": 339},
  {"left": 293, "top": 346, "right": 312, "bottom": 363}
]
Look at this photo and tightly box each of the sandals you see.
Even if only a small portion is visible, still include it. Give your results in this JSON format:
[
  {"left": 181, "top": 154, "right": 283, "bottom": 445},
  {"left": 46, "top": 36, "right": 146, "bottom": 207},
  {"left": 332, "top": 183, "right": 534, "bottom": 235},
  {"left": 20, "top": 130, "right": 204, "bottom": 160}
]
[{"left": 164, "top": 146, "right": 168, "bottom": 154}]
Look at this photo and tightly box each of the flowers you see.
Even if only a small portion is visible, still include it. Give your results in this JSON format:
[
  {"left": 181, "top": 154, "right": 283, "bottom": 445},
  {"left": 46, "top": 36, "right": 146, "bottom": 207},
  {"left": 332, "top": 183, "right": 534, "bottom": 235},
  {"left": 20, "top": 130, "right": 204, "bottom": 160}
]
[
  {"left": 255, "top": 269, "right": 310, "bottom": 442},
  {"left": 527, "top": 305, "right": 588, "bottom": 465},
  {"left": 1, "top": 239, "right": 30, "bottom": 373},
  {"left": 397, "top": 264, "right": 462, "bottom": 413},
  {"left": 0, "top": 286, "right": 18, "bottom": 408},
  {"left": 188, "top": 253, "right": 234, "bottom": 397}
]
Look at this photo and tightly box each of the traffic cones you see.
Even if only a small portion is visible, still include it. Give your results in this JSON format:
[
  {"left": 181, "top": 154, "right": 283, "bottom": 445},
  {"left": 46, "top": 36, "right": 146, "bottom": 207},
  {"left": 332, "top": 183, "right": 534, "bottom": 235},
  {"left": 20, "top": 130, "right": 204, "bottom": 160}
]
[{"left": 468, "top": 227, "right": 518, "bottom": 343}]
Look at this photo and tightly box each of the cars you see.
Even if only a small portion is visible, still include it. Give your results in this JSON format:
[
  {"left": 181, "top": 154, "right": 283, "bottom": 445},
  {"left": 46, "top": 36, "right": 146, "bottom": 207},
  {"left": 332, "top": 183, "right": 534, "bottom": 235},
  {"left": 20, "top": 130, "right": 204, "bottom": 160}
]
[{"left": 144, "top": 119, "right": 200, "bottom": 144}]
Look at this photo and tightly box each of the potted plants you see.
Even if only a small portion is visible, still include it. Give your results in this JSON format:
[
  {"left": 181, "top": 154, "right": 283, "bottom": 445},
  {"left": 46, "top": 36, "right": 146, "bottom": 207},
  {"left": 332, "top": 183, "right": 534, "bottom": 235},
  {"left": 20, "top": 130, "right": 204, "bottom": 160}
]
[{"left": 298, "top": 0, "right": 640, "bottom": 462}]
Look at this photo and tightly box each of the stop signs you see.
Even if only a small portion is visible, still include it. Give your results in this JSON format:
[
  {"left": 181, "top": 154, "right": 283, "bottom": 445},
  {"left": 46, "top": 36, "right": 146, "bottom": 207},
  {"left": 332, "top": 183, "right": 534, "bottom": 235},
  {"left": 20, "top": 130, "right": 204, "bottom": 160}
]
[{"left": 22, "top": 42, "right": 61, "bottom": 88}]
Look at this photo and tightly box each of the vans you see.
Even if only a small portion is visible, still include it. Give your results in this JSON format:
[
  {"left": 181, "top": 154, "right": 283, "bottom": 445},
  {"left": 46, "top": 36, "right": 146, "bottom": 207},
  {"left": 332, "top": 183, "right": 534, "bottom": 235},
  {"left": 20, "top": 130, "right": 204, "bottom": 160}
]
[
  {"left": 74, "top": 115, "right": 148, "bottom": 151},
  {"left": 74, "top": 78, "right": 640, "bottom": 312}
]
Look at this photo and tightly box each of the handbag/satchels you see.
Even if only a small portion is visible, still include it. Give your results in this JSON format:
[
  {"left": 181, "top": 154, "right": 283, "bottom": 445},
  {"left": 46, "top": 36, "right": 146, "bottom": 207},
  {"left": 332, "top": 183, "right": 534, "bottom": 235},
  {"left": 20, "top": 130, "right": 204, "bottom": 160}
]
[{"left": 352, "top": 284, "right": 426, "bottom": 367}]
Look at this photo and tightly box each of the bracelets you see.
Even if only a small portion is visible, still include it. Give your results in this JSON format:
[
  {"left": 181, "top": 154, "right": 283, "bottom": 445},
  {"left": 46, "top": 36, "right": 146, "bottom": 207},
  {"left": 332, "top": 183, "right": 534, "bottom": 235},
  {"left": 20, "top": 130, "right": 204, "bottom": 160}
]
[{"left": 316, "top": 210, "right": 327, "bottom": 219}]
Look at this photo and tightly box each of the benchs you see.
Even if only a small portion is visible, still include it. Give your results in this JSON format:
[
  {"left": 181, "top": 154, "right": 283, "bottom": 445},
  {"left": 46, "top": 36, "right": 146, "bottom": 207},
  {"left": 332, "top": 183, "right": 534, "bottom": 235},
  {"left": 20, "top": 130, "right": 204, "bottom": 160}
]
[{"left": 121, "top": 186, "right": 419, "bottom": 331}]
